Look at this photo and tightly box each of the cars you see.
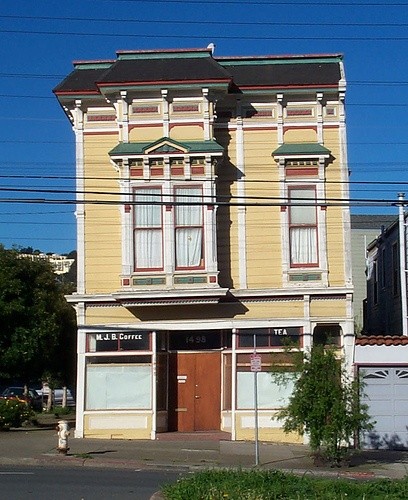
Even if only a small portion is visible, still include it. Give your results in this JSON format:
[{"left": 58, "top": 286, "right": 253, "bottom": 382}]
[{"left": 0, "top": 386, "right": 44, "bottom": 414}]
